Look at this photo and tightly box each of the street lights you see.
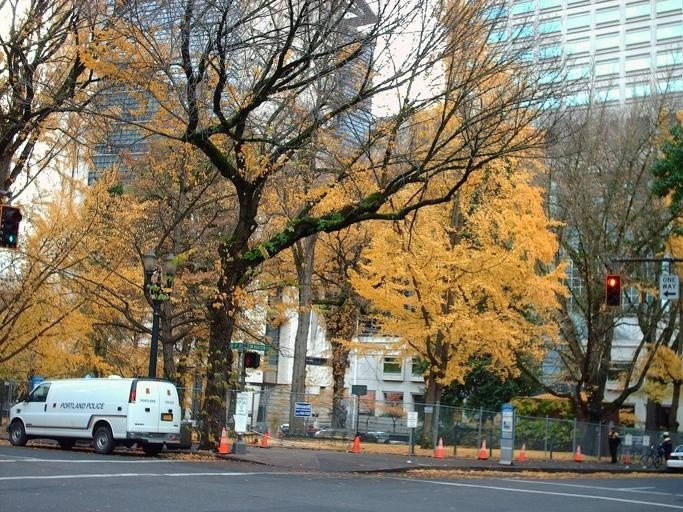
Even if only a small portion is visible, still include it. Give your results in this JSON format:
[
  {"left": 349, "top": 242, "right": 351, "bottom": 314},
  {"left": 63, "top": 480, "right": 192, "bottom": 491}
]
[
  {"left": 139, "top": 249, "right": 178, "bottom": 380},
  {"left": 249, "top": 389, "right": 267, "bottom": 430},
  {"left": 0, "top": 204, "right": 24, "bottom": 250}
]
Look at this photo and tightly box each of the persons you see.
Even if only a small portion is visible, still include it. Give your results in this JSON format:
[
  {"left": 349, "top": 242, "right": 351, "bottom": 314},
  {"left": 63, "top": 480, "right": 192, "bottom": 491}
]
[
  {"left": 607, "top": 425, "right": 623, "bottom": 465},
  {"left": 657, "top": 431, "right": 673, "bottom": 464}
]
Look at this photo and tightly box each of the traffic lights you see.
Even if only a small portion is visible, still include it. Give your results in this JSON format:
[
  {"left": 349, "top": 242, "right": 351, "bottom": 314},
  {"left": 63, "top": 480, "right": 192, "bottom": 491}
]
[
  {"left": 604, "top": 273, "right": 621, "bottom": 307},
  {"left": 242, "top": 351, "right": 261, "bottom": 368}
]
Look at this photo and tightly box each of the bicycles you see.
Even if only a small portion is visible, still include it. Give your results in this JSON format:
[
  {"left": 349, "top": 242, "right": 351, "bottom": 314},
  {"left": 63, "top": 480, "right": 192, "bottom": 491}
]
[{"left": 637, "top": 442, "right": 662, "bottom": 468}]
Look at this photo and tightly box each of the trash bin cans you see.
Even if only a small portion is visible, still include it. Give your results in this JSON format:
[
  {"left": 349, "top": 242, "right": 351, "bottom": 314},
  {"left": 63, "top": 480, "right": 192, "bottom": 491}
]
[{"left": 166, "top": 422, "right": 192, "bottom": 451}]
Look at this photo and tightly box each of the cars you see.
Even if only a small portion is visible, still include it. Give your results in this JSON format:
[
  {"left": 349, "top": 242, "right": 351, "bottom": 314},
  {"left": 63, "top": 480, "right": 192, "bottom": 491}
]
[
  {"left": 277, "top": 423, "right": 390, "bottom": 443},
  {"left": 665, "top": 444, "right": 683, "bottom": 472}
]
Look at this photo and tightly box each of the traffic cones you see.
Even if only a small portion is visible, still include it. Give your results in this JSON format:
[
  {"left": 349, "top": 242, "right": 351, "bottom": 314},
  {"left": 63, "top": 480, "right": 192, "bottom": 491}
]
[
  {"left": 621, "top": 450, "right": 630, "bottom": 463},
  {"left": 215, "top": 426, "right": 232, "bottom": 456},
  {"left": 258, "top": 432, "right": 268, "bottom": 449},
  {"left": 348, "top": 436, "right": 359, "bottom": 454},
  {"left": 432, "top": 436, "right": 444, "bottom": 461},
  {"left": 477, "top": 440, "right": 488, "bottom": 460},
  {"left": 573, "top": 444, "right": 585, "bottom": 462},
  {"left": 515, "top": 443, "right": 527, "bottom": 463}
]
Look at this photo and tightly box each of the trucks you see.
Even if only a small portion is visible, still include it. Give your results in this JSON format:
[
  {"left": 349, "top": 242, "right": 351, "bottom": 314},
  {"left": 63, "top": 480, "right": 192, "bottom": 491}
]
[{"left": 5, "top": 374, "right": 183, "bottom": 456}]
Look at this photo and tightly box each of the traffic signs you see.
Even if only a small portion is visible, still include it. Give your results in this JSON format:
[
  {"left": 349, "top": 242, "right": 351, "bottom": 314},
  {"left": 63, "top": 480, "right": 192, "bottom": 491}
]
[
  {"left": 294, "top": 402, "right": 312, "bottom": 418},
  {"left": 658, "top": 274, "right": 678, "bottom": 301},
  {"left": 227, "top": 342, "right": 271, "bottom": 352}
]
